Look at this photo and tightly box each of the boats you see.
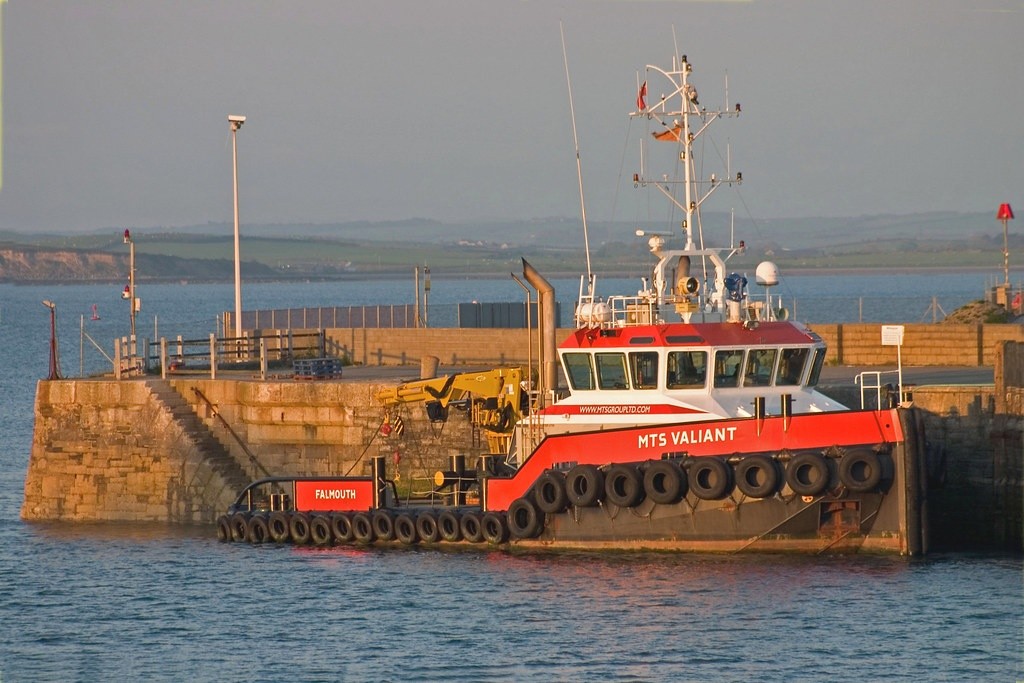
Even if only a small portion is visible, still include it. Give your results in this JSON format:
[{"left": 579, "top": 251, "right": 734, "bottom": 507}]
[{"left": 216, "top": 23, "right": 946, "bottom": 559}]
